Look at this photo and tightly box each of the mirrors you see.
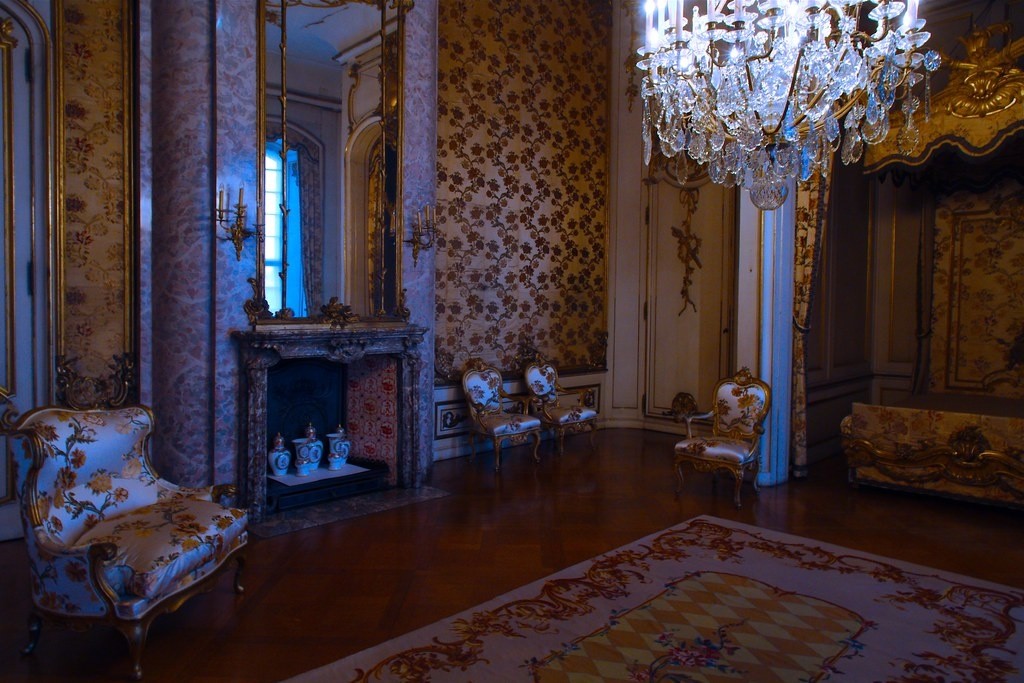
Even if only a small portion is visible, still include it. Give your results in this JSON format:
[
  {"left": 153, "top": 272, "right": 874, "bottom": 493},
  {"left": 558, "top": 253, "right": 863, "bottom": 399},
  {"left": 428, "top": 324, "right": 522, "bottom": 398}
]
[{"left": 258, "top": 0, "right": 406, "bottom": 326}]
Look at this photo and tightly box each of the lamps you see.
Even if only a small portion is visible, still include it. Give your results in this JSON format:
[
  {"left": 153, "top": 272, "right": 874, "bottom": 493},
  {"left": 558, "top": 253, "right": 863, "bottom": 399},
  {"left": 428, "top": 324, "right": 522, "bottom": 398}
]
[{"left": 636, "top": 0, "right": 941, "bottom": 210}]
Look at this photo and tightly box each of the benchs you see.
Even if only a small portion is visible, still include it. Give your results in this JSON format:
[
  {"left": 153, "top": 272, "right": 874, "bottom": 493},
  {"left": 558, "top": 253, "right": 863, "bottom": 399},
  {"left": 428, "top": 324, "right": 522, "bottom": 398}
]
[{"left": 0, "top": 352, "right": 263, "bottom": 683}]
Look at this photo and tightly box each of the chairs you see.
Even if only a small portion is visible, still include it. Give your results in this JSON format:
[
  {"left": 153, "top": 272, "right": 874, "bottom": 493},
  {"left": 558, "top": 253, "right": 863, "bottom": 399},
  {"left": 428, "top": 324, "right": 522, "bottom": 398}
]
[
  {"left": 515, "top": 330, "right": 602, "bottom": 465},
  {"left": 671, "top": 367, "right": 773, "bottom": 504},
  {"left": 457, "top": 356, "right": 544, "bottom": 470}
]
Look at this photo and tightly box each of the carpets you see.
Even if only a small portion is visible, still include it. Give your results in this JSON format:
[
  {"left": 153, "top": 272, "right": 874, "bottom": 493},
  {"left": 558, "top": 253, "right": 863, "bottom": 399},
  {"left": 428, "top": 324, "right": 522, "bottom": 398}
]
[{"left": 283, "top": 514, "right": 1024, "bottom": 683}]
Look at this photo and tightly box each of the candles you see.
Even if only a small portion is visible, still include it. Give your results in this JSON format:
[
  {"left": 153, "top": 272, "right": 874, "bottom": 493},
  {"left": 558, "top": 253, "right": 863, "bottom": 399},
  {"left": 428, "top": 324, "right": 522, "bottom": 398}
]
[
  {"left": 417, "top": 209, "right": 422, "bottom": 233},
  {"left": 219, "top": 190, "right": 223, "bottom": 211},
  {"left": 425, "top": 203, "right": 430, "bottom": 220},
  {"left": 433, "top": 209, "right": 437, "bottom": 225},
  {"left": 257, "top": 204, "right": 262, "bottom": 225},
  {"left": 239, "top": 188, "right": 243, "bottom": 206}
]
